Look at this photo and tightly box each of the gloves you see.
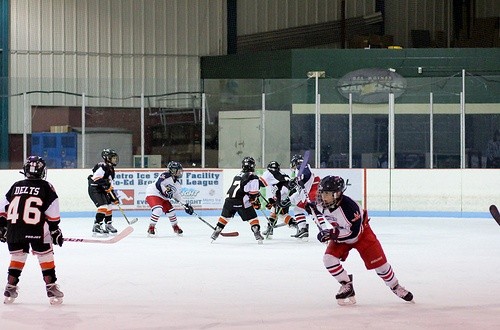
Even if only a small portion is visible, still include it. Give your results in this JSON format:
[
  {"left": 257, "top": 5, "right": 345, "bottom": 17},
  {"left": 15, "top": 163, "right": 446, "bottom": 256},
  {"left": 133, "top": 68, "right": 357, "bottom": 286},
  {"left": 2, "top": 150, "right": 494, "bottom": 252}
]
[
  {"left": 112, "top": 197, "right": 119, "bottom": 205},
  {"left": 317, "top": 228, "right": 340, "bottom": 242},
  {"left": 164, "top": 189, "right": 173, "bottom": 198},
  {"left": 304, "top": 203, "right": 318, "bottom": 215},
  {"left": 184, "top": 203, "right": 194, "bottom": 215},
  {"left": 49, "top": 225, "right": 64, "bottom": 247},
  {"left": 101, "top": 180, "right": 112, "bottom": 192},
  {"left": 0, "top": 212, "right": 8, "bottom": 243},
  {"left": 250, "top": 192, "right": 291, "bottom": 209},
  {"left": 288, "top": 177, "right": 301, "bottom": 190}
]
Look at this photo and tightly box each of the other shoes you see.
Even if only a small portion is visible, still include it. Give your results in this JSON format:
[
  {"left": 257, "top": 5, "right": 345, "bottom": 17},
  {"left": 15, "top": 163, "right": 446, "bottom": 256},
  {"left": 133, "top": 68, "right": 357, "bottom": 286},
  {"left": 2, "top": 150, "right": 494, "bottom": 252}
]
[
  {"left": 291, "top": 224, "right": 299, "bottom": 237},
  {"left": 263, "top": 225, "right": 274, "bottom": 238}
]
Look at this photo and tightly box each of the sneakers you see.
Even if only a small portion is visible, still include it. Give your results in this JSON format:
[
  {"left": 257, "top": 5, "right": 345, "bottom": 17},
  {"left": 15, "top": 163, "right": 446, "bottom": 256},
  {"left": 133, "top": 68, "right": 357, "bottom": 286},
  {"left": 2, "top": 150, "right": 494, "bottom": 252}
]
[
  {"left": 335, "top": 274, "right": 358, "bottom": 306},
  {"left": 209, "top": 231, "right": 221, "bottom": 243},
  {"left": 172, "top": 224, "right": 184, "bottom": 236},
  {"left": 391, "top": 284, "right": 414, "bottom": 304},
  {"left": 295, "top": 224, "right": 309, "bottom": 241},
  {"left": 3, "top": 284, "right": 18, "bottom": 304},
  {"left": 250, "top": 225, "right": 264, "bottom": 245},
  {"left": 92, "top": 221, "right": 117, "bottom": 237},
  {"left": 46, "top": 283, "right": 65, "bottom": 304},
  {"left": 147, "top": 224, "right": 157, "bottom": 238}
]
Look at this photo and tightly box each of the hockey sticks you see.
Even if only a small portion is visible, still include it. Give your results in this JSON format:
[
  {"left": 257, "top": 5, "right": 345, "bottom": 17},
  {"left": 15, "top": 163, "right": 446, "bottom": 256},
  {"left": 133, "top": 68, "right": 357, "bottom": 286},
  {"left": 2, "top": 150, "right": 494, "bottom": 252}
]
[
  {"left": 296, "top": 151, "right": 328, "bottom": 246},
  {"left": 259, "top": 206, "right": 289, "bottom": 228},
  {"left": 489, "top": 204, "right": 500, "bottom": 225},
  {"left": 62, "top": 226, "right": 135, "bottom": 245},
  {"left": 174, "top": 197, "right": 239, "bottom": 237},
  {"left": 265, "top": 207, "right": 284, "bottom": 239},
  {"left": 108, "top": 188, "right": 138, "bottom": 225}
]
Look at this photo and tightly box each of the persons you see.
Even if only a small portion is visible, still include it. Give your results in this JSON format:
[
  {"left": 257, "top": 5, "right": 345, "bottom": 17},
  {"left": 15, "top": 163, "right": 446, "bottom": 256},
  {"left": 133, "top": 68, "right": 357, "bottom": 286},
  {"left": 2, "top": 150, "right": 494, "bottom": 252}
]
[
  {"left": 145, "top": 162, "right": 194, "bottom": 234},
  {"left": 211, "top": 155, "right": 321, "bottom": 240},
  {"left": 86, "top": 148, "right": 120, "bottom": 233},
  {"left": 487, "top": 130, "right": 500, "bottom": 168},
  {"left": 304, "top": 175, "right": 414, "bottom": 301},
  {"left": 134, "top": 139, "right": 148, "bottom": 155},
  {"left": 0, "top": 156, "right": 64, "bottom": 298}
]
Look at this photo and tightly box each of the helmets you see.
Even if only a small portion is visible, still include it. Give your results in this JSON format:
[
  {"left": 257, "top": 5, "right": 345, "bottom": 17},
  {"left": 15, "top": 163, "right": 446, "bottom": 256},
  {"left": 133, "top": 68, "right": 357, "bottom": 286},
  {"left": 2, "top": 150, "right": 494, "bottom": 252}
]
[
  {"left": 166, "top": 161, "right": 183, "bottom": 172},
  {"left": 100, "top": 149, "right": 119, "bottom": 166},
  {"left": 241, "top": 156, "right": 256, "bottom": 173},
  {"left": 21, "top": 156, "right": 47, "bottom": 179},
  {"left": 317, "top": 175, "right": 347, "bottom": 206},
  {"left": 290, "top": 154, "right": 306, "bottom": 169},
  {"left": 267, "top": 161, "right": 281, "bottom": 173}
]
